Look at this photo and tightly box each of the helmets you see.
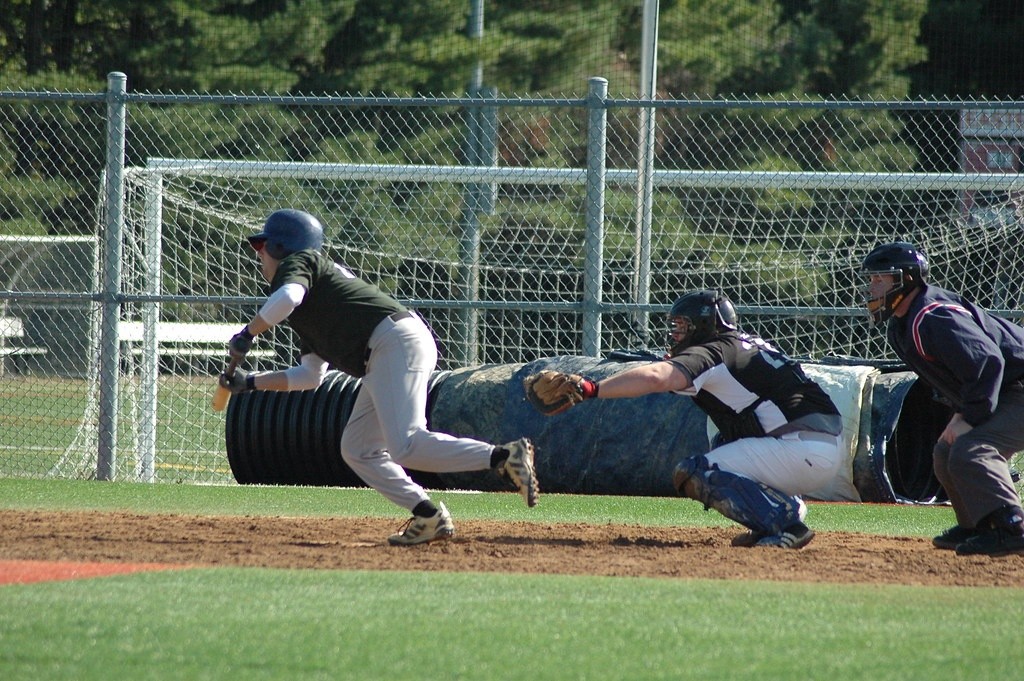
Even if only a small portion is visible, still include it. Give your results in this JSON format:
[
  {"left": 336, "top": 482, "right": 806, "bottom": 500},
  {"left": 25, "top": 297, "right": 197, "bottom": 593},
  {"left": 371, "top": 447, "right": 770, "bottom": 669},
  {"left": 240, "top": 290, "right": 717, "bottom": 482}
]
[
  {"left": 248, "top": 208, "right": 322, "bottom": 260},
  {"left": 667, "top": 290, "right": 737, "bottom": 355},
  {"left": 859, "top": 243, "right": 928, "bottom": 322}
]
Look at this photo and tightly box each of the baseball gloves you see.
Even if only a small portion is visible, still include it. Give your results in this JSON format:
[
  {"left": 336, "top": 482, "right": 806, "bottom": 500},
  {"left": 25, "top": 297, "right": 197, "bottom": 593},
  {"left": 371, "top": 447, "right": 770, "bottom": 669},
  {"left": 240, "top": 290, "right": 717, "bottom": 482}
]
[{"left": 521, "top": 367, "right": 593, "bottom": 417}]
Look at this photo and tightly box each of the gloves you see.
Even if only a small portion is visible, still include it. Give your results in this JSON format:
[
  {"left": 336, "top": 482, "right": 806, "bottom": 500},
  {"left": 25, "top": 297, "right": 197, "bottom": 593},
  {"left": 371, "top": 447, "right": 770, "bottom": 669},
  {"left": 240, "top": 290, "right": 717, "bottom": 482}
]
[
  {"left": 229, "top": 326, "right": 254, "bottom": 363},
  {"left": 219, "top": 367, "right": 257, "bottom": 392}
]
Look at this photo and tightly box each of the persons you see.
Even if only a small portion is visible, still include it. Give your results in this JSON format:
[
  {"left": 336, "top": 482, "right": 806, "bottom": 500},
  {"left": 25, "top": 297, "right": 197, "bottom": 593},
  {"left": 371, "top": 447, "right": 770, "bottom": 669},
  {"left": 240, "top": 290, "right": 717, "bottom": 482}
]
[
  {"left": 218, "top": 208, "right": 540, "bottom": 546},
  {"left": 860, "top": 242, "right": 1024, "bottom": 557},
  {"left": 523, "top": 289, "right": 843, "bottom": 549}
]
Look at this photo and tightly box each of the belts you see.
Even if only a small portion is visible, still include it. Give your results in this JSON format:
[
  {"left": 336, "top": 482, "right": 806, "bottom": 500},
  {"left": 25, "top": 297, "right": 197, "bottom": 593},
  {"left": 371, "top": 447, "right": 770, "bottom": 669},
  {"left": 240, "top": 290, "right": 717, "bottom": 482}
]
[
  {"left": 777, "top": 430, "right": 837, "bottom": 445},
  {"left": 363, "top": 311, "right": 414, "bottom": 372}
]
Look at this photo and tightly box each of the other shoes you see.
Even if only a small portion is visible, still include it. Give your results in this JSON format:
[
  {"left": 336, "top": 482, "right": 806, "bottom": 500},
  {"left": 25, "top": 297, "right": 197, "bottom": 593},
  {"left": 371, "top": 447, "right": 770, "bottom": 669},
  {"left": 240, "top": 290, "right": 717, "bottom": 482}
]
[
  {"left": 955, "top": 506, "right": 1024, "bottom": 554},
  {"left": 931, "top": 523, "right": 994, "bottom": 549}
]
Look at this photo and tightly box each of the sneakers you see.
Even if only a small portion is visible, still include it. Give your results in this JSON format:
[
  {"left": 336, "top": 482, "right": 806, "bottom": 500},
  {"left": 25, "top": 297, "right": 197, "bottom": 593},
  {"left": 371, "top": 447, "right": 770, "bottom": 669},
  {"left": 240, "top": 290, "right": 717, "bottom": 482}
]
[
  {"left": 755, "top": 520, "right": 815, "bottom": 550},
  {"left": 495, "top": 437, "right": 539, "bottom": 507},
  {"left": 387, "top": 501, "right": 454, "bottom": 546},
  {"left": 732, "top": 527, "right": 768, "bottom": 547}
]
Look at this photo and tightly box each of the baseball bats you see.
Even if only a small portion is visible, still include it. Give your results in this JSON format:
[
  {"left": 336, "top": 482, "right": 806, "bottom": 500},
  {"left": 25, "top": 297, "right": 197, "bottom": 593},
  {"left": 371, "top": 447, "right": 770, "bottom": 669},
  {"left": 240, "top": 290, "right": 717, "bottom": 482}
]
[{"left": 211, "top": 354, "right": 239, "bottom": 412}]
